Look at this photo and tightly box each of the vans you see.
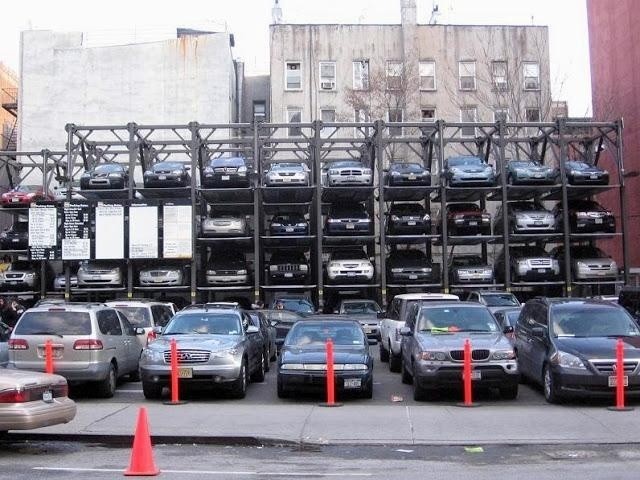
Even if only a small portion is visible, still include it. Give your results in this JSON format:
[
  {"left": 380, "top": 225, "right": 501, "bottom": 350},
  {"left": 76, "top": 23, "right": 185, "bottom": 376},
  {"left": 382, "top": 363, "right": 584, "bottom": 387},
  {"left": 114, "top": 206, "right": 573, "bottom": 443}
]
[
  {"left": 9, "top": 306, "right": 144, "bottom": 401},
  {"left": 109, "top": 302, "right": 175, "bottom": 346},
  {"left": 515, "top": 296, "right": 639, "bottom": 406}
]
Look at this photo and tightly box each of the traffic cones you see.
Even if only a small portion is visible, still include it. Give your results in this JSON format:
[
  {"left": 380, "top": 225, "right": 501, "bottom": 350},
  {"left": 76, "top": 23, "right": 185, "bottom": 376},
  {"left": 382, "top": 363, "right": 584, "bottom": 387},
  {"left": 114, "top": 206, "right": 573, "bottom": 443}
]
[{"left": 120, "top": 407, "right": 170, "bottom": 477}]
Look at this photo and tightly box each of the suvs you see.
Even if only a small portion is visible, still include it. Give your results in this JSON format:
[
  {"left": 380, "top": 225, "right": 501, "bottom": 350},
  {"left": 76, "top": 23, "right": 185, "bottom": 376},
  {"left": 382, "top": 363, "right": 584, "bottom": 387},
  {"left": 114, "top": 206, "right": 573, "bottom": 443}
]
[
  {"left": 402, "top": 301, "right": 520, "bottom": 402},
  {"left": 139, "top": 305, "right": 263, "bottom": 399}
]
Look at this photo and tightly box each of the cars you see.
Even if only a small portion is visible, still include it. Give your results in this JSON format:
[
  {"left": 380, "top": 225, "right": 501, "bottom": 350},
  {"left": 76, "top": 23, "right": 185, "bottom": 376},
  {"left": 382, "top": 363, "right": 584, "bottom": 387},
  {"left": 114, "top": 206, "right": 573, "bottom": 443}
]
[
  {"left": 261, "top": 312, "right": 298, "bottom": 343},
  {"left": 333, "top": 298, "right": 382, "bottom": 343},
  {"left": 276, "top": 297, "right": 318, "bottom": 314},
  {"left": 1, "top": 370, "right": 77, "bottom": 430},
  {"left": 0, "top": 321, "right": 13, "bottom": 367},
  {"left": 465, "top": 291, "right": 525, "bottom": 316},
  {"left": 278, "top": 320, "right": 373, "bottom": 403}
]
